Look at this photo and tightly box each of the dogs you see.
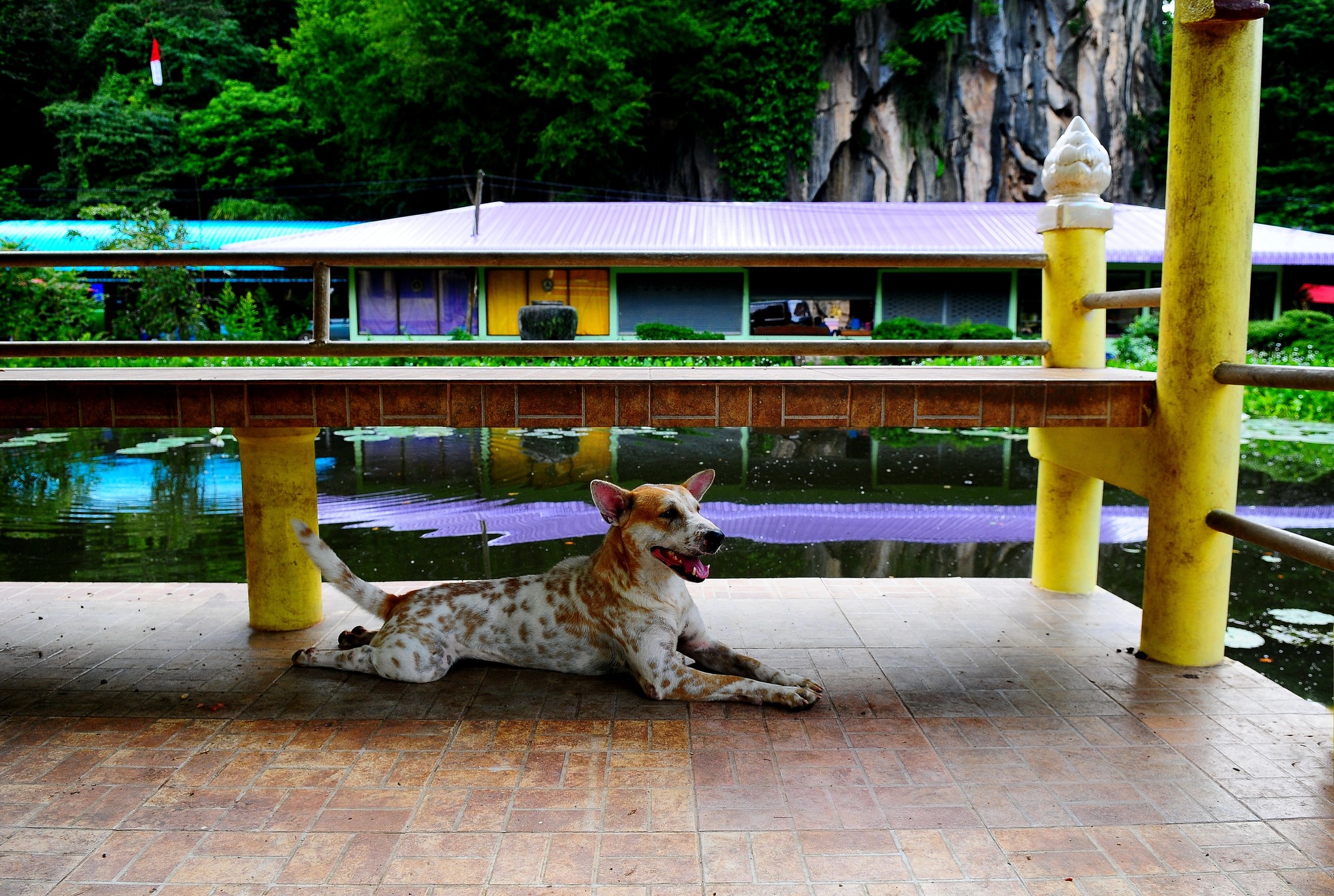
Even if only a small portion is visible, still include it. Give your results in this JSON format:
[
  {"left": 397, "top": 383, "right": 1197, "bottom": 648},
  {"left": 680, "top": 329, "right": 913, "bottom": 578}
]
[{"left": 290, "top": 469, "right": 823, "bottom": 710}]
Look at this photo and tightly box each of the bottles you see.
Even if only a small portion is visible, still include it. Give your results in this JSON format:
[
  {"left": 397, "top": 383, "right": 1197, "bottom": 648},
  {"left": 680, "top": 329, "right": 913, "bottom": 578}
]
[{"left": 518, "top": 300, "right": 578, "bottom": 340}]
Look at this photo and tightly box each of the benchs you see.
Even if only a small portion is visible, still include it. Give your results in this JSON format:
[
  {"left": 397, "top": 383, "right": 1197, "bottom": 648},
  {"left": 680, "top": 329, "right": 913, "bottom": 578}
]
[{"left": 0, "top": 365, "right": 1159, "bottom": 634}]
